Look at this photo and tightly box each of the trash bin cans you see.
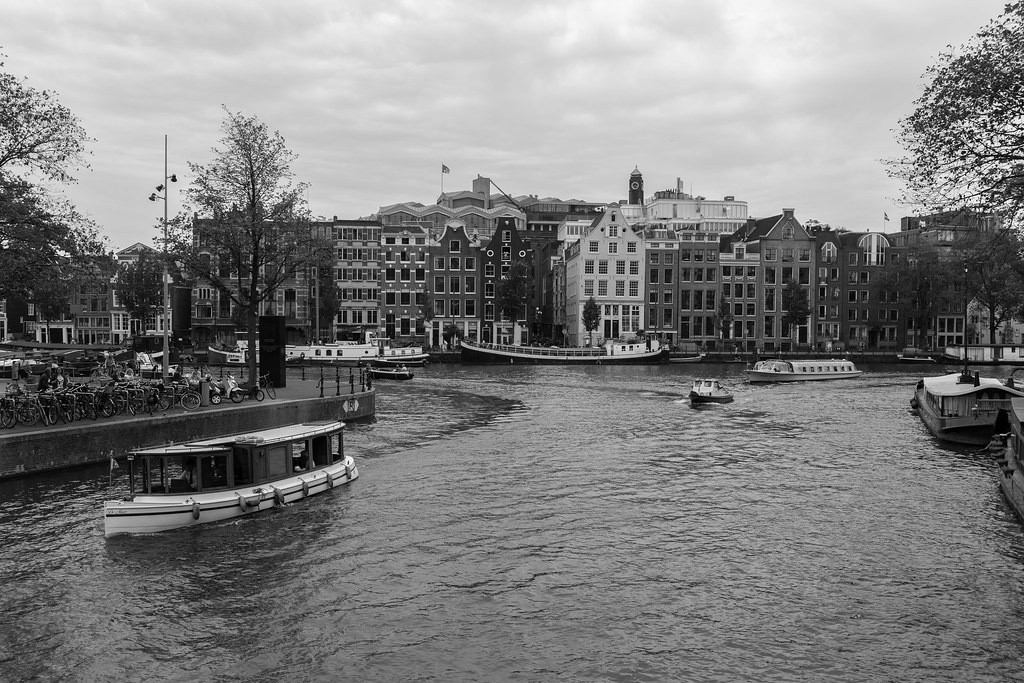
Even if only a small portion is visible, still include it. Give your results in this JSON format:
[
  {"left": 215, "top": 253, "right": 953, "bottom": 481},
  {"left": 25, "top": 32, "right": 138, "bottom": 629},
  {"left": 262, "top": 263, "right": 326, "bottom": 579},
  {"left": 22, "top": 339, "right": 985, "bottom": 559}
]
[{"left": 12, "top": 362, "right": 20, "bottom": 380}]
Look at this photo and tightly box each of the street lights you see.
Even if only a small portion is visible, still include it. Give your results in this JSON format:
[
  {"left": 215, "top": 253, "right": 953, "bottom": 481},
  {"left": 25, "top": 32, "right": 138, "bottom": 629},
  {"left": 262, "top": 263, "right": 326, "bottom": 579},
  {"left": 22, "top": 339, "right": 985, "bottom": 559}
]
[{"left": 148, "top": 134, "right": 177, "bottom": 381}]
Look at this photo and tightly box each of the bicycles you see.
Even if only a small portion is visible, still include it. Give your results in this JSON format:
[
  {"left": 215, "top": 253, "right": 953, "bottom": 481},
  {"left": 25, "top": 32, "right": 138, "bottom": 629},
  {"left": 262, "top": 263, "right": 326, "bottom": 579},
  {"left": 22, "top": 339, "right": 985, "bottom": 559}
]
[{"left": 0, "top": 355, "right": 277, "bottom": 428}]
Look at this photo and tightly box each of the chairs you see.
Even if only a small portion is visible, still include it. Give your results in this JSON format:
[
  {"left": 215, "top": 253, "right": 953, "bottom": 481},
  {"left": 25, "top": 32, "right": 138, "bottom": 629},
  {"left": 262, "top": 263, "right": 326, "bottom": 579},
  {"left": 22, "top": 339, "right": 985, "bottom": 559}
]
[{"left": 170, "top": 478, "right": 189, "bottom": 493}]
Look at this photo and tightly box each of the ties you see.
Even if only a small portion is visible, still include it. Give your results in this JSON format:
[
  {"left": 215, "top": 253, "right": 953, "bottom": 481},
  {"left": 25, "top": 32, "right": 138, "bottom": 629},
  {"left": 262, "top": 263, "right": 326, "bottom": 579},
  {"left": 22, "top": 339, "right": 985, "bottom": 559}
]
[{"left": 189, "top": 471, "right": 193, "bottom": 485}]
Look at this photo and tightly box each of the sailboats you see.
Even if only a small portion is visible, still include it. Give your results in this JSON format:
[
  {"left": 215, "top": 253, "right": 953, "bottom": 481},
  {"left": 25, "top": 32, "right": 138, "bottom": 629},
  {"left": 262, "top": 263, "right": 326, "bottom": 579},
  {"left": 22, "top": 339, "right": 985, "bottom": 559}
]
[{"left": 909, "top": 251, "right": 1024, "bottom": 446}]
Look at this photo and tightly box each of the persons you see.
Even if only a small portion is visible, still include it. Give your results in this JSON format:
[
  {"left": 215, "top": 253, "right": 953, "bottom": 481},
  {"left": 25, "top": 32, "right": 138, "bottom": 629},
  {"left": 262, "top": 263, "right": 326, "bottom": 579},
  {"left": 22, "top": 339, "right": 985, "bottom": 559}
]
[
  {"left": 305, "top": 338, "right": 310, "bottom": 346},
  {"left": 301, "top": 450, "right": 315, "bottom": 472},
  {"left": 107, "top": 353, "right": 134, "bottom": 381},
  {"left": 734, "top": 344, "right": 741, "bottom": 361},
  {"left": 178, "top": 459, "right": 198, "bottom": 488},
  {"left": 319, "top": 339, "right": 322, "bottom": 346},
  {"left": 465, "top": 336, "right": 470, "bottom": 345},
  {"left": 173, "top": 366, "right": 201, "bottom": 385},
  {"left": 70, "top": 339, "right": 77, "bottom": 344},
  {"left": 482, "top": 338, "right": 487, "bottom": 348},
  {"left": 36, "top": 356, "right": 75, "bottom": 391},
  {"left": 396, "top": 364, "right": 407, "bottom": 372}
]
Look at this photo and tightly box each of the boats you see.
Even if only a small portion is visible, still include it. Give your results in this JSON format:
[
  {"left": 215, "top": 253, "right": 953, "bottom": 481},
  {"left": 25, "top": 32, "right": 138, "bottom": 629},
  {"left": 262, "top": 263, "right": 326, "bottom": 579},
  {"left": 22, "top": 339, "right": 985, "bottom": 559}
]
[
  {"left": 104, "top": 419, "right": 360, "bottom": 540},
  {"left": 207, "top": 339, "right": 433, "bottom": 369},
  {"left": 987, "top": 397, "right": 1024, "bottom": 521},
  {"left": 460, "top": 333, "right": 671, "bottom": 366},
  {"left": 745, "top": 358, "right": 863, "bottom": 382},
  {"left": 688, "top": 378, "right": 735, "bottom": 404},
  {"left": 362, "top": 366, "right": 416, "bottom": 380},
  {"left": 899, "top": 356, "right": 937, "bottom": 364}
]
